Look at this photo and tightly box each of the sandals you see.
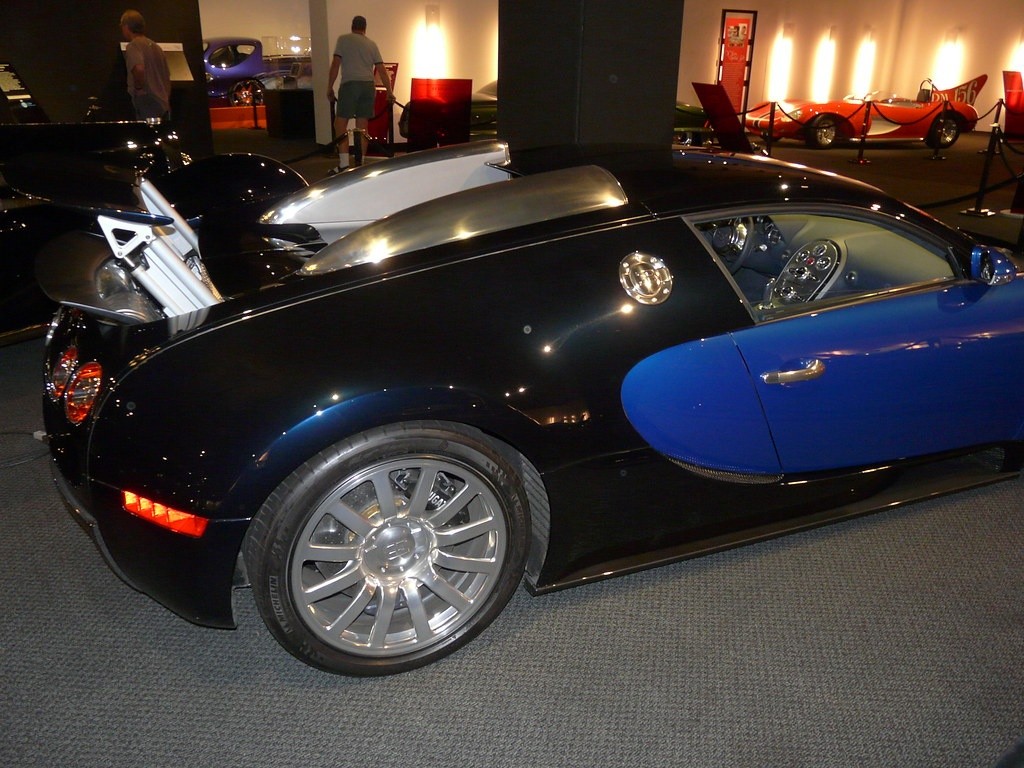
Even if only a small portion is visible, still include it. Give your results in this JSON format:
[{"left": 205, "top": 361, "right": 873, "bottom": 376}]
[{"left": 327, "top": 166, "right": 348, "bottom": 175}]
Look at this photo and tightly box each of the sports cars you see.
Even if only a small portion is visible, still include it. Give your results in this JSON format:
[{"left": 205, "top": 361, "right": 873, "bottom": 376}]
[
  {"left": 0, "top": 115, "right": 1024, "bottom": 677},
  {"left": 201, "top": 37, "right": 313, "bottom": 106},
  {"left": 398, "top": 79, "right": 498, "bottom": 142},
  {"left": 745, "top": 78, "right": 978, "bottom": 150}
]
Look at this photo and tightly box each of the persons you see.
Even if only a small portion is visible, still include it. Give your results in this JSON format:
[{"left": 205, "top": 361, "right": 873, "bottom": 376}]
[
  {"left": 120, "top": 9, "right": 172, "bottom": 123},
  {"left": 327, "top": 16, "right": 397, "bottom": 178}
]
